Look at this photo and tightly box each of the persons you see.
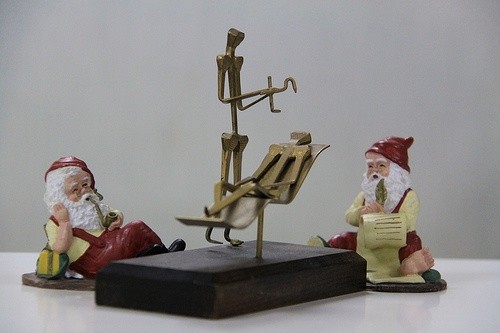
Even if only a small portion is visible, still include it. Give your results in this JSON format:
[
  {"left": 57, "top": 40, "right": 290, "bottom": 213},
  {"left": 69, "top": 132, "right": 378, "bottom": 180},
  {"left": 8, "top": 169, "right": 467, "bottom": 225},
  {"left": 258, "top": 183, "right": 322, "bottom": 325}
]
[
  {"left": 43, "top": 154, "right": 186, "bottom": 281},
  {"left": 306, "top": 135, "right": 436, "bottom": 276}
]
